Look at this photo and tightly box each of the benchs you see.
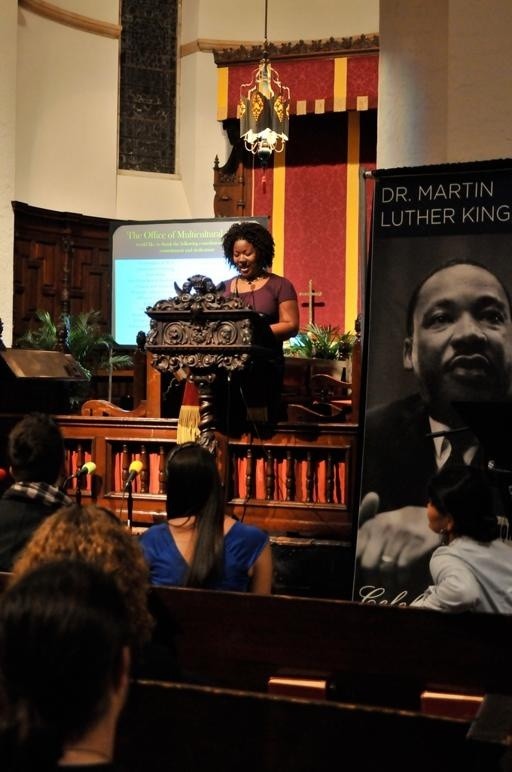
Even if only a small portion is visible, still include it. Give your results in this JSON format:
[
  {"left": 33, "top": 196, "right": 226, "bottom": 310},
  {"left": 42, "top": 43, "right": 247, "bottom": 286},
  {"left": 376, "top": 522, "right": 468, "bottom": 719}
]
[{"left": 1, "top": 566, "right": 510, "bottom": 771}]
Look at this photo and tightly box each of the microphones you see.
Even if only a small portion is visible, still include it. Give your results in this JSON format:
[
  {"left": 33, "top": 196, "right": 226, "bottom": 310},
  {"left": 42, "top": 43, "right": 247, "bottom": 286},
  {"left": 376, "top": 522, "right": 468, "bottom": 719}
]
[
  {"left": 125, "top": 460, "right": 143, "bottom": 488},
  {"left": 76, "top": 461, "right": 96, "bottom": 477}
]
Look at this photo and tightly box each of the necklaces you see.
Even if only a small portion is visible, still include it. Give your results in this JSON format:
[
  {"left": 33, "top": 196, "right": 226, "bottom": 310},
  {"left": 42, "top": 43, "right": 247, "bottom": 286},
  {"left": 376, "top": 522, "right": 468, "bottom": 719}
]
[
  {"left": 64, "top": 745, "right": 112, "bottom": 760},
  {"left": 239, "top": 270, "right": 266, "bottom": 285}
]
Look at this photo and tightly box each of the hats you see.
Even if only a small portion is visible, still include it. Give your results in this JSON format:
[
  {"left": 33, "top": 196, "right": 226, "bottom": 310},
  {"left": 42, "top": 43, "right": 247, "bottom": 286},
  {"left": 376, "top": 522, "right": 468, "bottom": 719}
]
[{"left": 417, "top": 462, "right": 502, "bottom": 542}]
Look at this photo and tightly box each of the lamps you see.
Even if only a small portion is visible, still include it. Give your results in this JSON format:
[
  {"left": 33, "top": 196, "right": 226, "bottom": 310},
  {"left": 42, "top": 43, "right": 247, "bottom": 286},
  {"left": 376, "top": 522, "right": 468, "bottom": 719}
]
[{"left": 236, "top": 0, "right": 291, "bottom": 196}]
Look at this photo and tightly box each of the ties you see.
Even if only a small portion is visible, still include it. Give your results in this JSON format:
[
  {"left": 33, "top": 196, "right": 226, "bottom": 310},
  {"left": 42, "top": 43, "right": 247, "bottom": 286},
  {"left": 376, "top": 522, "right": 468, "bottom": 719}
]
[{"left": 440, "top": 432, "right": 470, "bottom": 474}]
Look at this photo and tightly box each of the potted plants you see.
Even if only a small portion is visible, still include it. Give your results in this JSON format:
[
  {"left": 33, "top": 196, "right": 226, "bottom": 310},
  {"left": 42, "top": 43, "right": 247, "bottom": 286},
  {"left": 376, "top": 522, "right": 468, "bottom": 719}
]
[{"left": 283, "top": 320, "right": 355, "bottom": 395}]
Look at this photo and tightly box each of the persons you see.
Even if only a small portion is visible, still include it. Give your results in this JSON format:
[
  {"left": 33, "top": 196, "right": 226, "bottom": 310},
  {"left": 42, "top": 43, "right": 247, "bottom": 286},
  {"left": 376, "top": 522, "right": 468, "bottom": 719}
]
[
  {"left": 137, "top": 441, "right": 273, "bottom": 596},
  {"left": 406, "top": 462, "right": 512, "bottom": 614},
  {"left": 1, "top": 412, "right": 77, "bottom": 571},
  {"left": 1, "top": 557, "right": 135, "bottom": 772},
  {"left": 350, "top": 256, "right": 512, "bottom": 606},
  {"left": 1, "top": 503, "right": 157, "bottom": 651},
  {"left": 210, "top": 222, "right": 300, "bottom": 440}
]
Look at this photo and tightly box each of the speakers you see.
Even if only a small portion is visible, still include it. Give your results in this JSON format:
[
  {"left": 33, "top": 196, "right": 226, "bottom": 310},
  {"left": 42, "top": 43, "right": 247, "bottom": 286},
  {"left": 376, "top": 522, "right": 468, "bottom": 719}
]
[{"left": 0, "top": 347, "right": 90, "bottom": 418}]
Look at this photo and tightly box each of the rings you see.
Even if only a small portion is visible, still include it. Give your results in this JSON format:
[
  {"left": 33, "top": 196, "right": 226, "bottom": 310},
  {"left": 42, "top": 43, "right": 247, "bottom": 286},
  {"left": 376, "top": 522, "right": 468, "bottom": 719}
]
[{"left": 382, "top": 555, "right": 395, "bottom": 564}]
